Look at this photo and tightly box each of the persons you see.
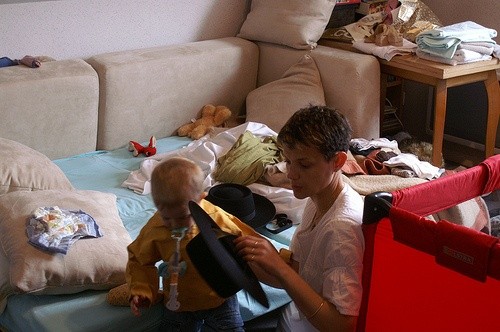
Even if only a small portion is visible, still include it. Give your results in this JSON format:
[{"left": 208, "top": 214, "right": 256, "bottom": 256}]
[
  {"left": 233, "top": 105, "right": 364, "bottom": 332},
  {"left": 126, "top": 158, "right": 299, "bottom": 332}
]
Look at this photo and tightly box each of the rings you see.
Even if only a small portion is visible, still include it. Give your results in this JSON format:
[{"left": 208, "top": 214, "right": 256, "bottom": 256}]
[
  {"left": 254, "top": 242, "right": 257, "bottom": 245},
  {"left": 251, "top": 248, "right": 254, "bottom": 253},
  {"left": 252, "top": 255, "right": 255, "bottom": 259}
]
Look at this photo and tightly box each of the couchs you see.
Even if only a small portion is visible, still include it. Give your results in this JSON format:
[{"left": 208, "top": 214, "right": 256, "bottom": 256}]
[{"left": 0, "top": 37, "right": 489, "bottom": 332}]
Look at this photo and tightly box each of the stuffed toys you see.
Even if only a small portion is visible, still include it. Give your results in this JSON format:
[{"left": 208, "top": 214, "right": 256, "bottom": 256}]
[{"left": 177, "top": 105, "right": 231, "bottom": 140}]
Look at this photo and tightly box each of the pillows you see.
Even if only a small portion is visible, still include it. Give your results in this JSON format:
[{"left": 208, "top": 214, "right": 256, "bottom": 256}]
[
  {"left": 236, "top": 0, "right": 336, "bottom": 50},
  {"left": 245, "top": 54, "right": 326, "bottom": 133},
  {"left": 0, "top": 190, "right": 133, "bottom": 294},
  {"left": 0, "top": 138, "right": 76, "bottom": 195}
]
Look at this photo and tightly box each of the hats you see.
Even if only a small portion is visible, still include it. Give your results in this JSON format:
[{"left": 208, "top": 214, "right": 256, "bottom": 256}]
[
  {"left": 206, "top": 182, "right": 277, "bottom": 229},
  {"left": 184, "top": 200, "right": 271, "bottom": 308}
]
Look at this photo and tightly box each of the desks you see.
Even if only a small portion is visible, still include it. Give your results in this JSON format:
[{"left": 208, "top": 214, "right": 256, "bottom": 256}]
[{"left": 323, "top": 28, "right": 500, "bottom": 170}]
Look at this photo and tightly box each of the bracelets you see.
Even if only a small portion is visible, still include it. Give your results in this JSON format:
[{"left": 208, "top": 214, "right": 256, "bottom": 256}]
[{"left": 305, "top": 303, "right": 324, "bottom": 318}]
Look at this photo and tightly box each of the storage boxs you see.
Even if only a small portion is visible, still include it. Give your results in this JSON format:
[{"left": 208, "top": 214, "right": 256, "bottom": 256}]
[{"left": 355, "top": 2, "right": 385, "bottom": 15}]
